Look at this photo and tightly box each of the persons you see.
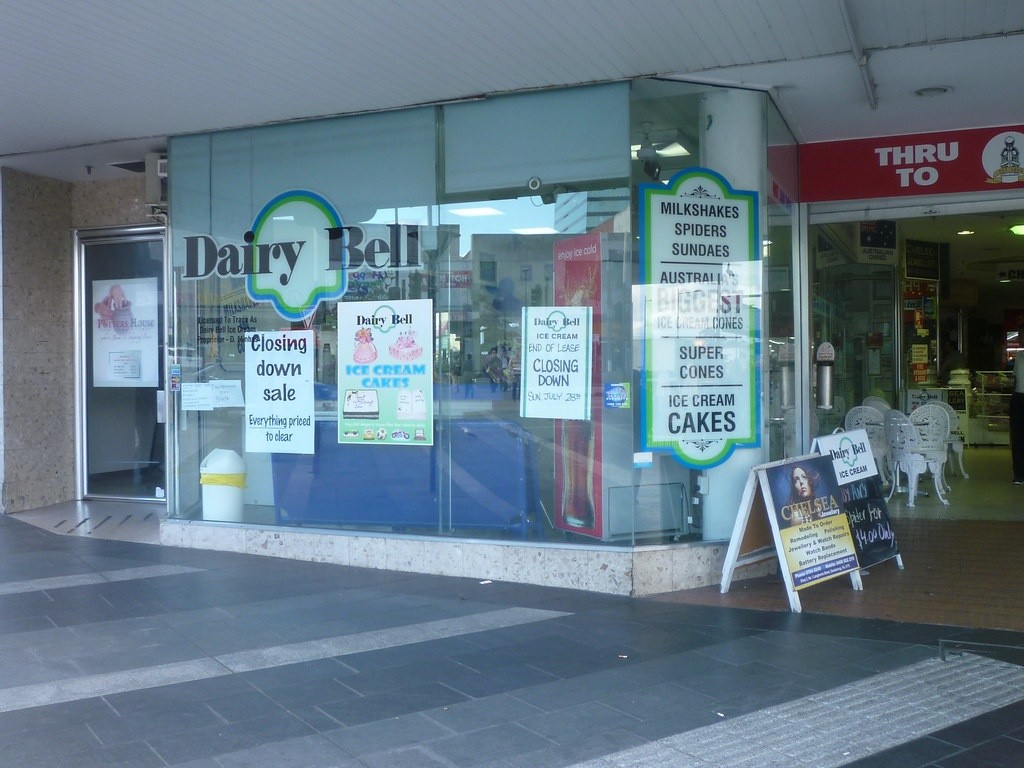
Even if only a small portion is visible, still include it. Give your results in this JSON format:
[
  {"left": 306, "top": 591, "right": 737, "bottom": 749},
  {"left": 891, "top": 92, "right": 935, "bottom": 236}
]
[
  {"left": 451, "top": 355, "right": 459, "bottom": 393},
  {"left": 943, "top": 340, "right": 962, "bottom": 386},
  {"left": 461, "top": 354, "right": 474, "bottom": 400},
  {"left": 485, "top": 348, "right": 501, "bottom": 394},
  {"left": 501, "top": 351, "right": 509, "bottom": 391},
  {"left": 788, "top": 466, "right": 827, "bottom": 527},
  {"left": 508, "top": 349, "right": 520, "bottom": 402},
  {"left": 1009, "top": 323, "right": 1024, "bottom": 485}
]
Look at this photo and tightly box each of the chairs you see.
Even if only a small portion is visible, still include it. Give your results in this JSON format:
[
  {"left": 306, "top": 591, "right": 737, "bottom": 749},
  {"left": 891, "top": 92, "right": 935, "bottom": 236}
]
[{"left": 844, "top": 396, "right": 969, "bottom": 508}]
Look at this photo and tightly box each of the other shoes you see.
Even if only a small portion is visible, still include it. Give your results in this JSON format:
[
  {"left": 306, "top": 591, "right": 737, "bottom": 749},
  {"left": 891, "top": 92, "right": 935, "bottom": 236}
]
[{"left": 1012, "top": 474, "right": 1024, "bottom": 485}]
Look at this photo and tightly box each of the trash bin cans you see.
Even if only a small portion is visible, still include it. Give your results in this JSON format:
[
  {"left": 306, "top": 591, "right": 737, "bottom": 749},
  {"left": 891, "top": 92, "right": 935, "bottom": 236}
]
[{"left": 199, "top": 448, "right": 246, "bottom": 522}]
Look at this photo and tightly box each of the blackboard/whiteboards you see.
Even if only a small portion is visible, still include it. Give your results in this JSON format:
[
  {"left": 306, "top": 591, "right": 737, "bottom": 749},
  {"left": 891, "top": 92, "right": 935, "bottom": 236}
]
[{"left": 816, "top": 427, "right": 901, "bottom": 570}]
